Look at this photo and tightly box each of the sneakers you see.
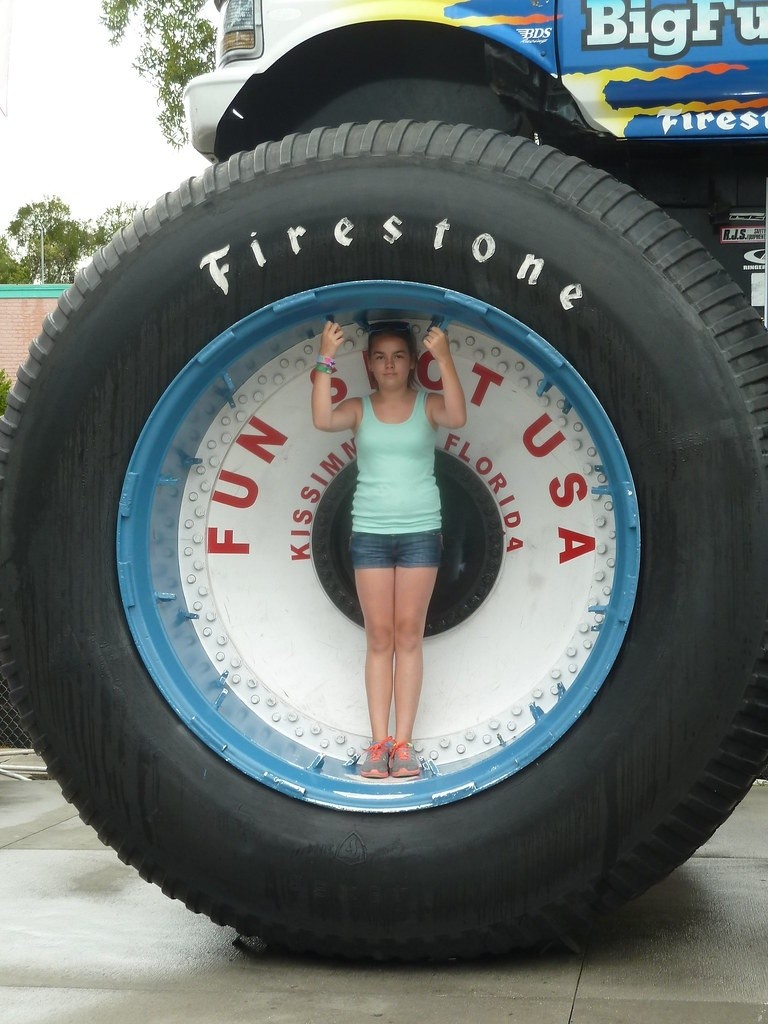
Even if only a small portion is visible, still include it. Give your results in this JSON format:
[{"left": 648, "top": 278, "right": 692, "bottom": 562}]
[
  {"left": 388, "top": 741, "right": 421, "bottom": 777},
  {"left": 362, "top": 734, "right": 394, "bottom": 778}
]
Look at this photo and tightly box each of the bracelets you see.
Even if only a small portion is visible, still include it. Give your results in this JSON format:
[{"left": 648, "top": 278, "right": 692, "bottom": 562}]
[
  {"left": 314, "top": 364, "right": 332, "bottom": 375},
  {"left": 316, "top": 354, "right": 336, "bottom": 369}
]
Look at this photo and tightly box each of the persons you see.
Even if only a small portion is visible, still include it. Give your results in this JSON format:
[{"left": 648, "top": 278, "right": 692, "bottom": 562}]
[{"left": 311, "top": 321, "right": 467, "bottom": 779}]
